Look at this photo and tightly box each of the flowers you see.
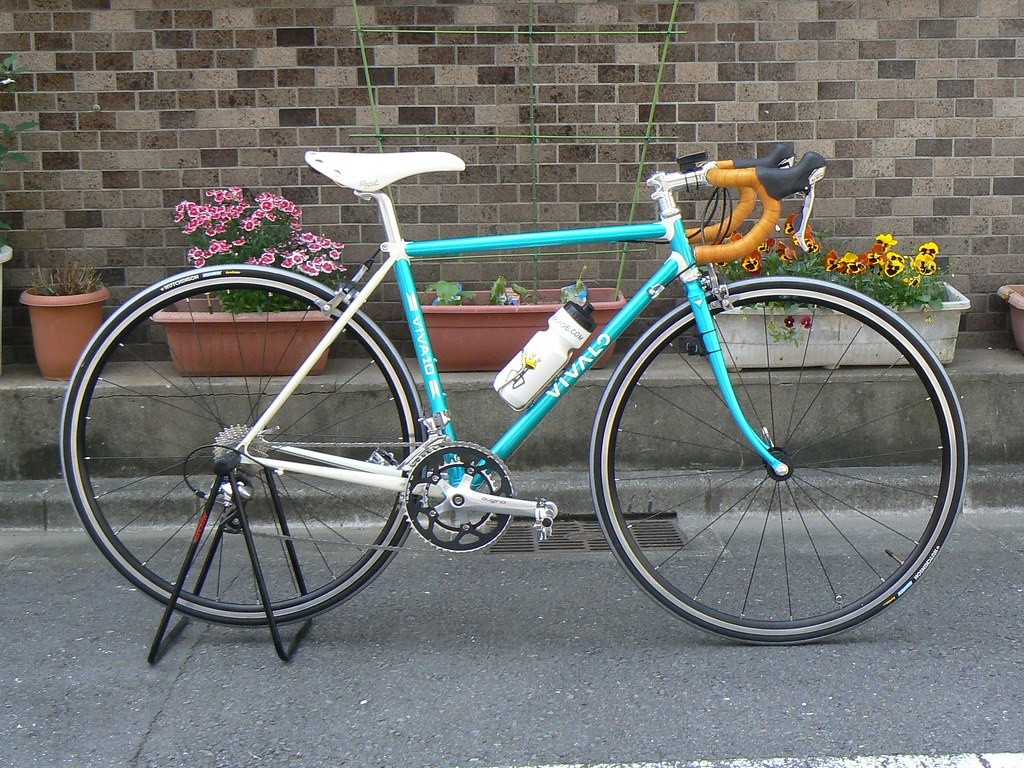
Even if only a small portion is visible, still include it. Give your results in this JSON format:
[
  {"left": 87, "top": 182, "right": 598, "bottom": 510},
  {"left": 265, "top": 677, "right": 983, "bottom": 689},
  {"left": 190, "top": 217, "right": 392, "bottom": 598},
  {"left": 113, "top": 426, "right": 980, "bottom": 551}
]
[
  {"left": 720, "top": 214, "right": 946, "bottom": 345},
  {"left": 176, "top": 185, "right": 349, "bottom": 314},
  {"left": 0, "top": 55, "right": 39, "bottom": 249}
]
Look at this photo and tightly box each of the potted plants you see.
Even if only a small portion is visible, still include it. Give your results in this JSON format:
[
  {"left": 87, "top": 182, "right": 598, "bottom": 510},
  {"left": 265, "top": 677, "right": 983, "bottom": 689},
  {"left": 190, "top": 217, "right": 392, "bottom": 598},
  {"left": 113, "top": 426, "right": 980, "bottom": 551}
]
[
  {"left": 19, "top": 257, "right": 110, "bottom": 379},
  {"left": 417, "top": 278, "right": 626, "bottom": 368}
]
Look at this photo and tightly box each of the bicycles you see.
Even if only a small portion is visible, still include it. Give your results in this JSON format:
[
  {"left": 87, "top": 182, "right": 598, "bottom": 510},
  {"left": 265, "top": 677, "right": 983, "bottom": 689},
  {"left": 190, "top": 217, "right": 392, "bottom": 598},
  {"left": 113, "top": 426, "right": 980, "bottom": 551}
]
[{"left": 55, "top": 149, "right": 968, "bottom": 648}]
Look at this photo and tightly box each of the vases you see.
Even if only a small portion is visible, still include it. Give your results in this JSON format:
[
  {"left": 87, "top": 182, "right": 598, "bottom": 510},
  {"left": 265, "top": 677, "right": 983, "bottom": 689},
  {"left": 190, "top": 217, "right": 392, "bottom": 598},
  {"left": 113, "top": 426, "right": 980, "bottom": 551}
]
[
  {"left": 995, "top": 284, "right": 1024, "bottom": 356},
  {"left": 0, "top": 244, "right": 13, "bottom": 302},
  {"left": 716, "top": 286, "right": 973, "bottom": 375},
  {"left": 150, "top": 297, "right": 335, "bottom": 376}
]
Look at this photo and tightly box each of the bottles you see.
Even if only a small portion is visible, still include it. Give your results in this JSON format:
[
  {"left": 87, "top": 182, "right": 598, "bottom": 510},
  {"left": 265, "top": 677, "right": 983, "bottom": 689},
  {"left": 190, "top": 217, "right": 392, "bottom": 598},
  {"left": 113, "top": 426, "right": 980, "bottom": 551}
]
[{"left": 494, "top": 301, "right": 599, "bottom": 410}]
